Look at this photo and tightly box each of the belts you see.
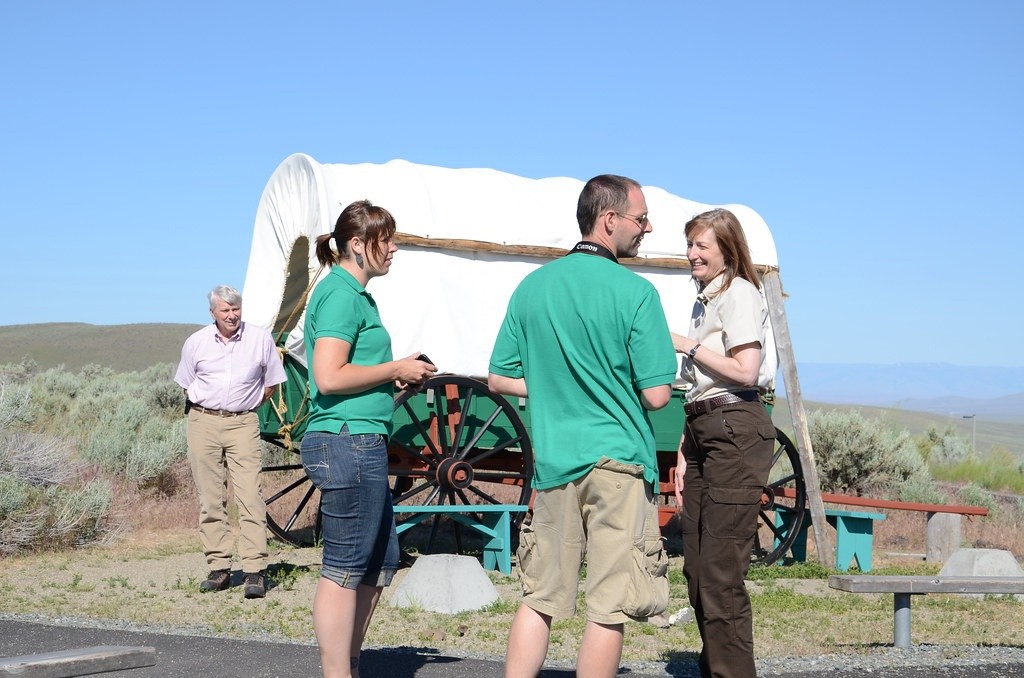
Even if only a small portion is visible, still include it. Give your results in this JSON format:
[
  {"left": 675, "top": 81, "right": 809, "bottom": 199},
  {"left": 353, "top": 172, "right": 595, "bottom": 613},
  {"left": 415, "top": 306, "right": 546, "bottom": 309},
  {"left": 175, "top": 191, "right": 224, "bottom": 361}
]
[
  {"left": 683, "top": 390, "right": 760, "bottom": 415},
  {"left": 190, "top": 403, "right": 251, "bottom": 418}
]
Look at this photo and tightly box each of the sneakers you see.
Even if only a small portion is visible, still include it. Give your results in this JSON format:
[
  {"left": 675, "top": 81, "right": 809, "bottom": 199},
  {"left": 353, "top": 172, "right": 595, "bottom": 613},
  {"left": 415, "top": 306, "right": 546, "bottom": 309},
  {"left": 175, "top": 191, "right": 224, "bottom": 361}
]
[
  {"left": 242, "top": 570, "right": 265, "bottom": 597},
  {"left": 200, "top": 569, "right": 231, "bottom": 591}
]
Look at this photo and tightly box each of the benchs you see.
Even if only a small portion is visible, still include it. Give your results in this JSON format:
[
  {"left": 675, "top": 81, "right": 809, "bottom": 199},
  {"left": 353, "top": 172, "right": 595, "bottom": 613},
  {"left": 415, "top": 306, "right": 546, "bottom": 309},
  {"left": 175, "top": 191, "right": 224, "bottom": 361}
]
[
  {"left": 828, "top": 575, "right": 1024, "bottom": 650},
  {"left": 391, "top": 505, "right": 530, "bottom": 574},
  {"left": 769, "top": 505, "right": 887, "bottom": 573}
]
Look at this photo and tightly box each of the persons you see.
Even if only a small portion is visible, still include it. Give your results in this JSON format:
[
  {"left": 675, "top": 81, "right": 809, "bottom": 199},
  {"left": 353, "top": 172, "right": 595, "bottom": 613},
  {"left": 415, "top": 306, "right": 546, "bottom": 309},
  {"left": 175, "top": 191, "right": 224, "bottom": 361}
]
[
  {"left": 670, "top": 208, "right": 778, "bottom": 678},
  {"left": 174, "top": 285, "right": 288, "bottom": 597},
  {"left": 299, "top": 200, "right": 437, "bottom": 678},
  {"left": 489, "top": 173, "right": 678, "bottom": 678}
]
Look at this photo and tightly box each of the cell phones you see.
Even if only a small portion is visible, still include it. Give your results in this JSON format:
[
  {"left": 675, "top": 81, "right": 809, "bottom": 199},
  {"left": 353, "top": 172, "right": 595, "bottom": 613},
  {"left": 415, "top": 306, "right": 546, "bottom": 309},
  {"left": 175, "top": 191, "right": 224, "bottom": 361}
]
[{"left": 406, "top": 354, "right": 434, "bottom": 389}]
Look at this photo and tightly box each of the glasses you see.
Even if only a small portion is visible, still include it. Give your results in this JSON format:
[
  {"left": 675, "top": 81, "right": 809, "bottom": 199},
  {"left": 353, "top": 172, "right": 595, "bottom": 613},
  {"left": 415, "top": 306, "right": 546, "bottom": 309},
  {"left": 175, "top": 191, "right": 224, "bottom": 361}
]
[{"left": 600, "top": 210, "right": 647, "bottom": 224}]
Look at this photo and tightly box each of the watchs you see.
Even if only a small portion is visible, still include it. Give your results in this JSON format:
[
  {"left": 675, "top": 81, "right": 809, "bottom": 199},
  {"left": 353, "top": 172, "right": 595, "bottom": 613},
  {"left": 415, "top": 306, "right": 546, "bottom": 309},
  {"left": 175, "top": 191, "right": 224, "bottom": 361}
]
[{"left": 689, "top": 344, "right": 701, "bottom": 359}]
